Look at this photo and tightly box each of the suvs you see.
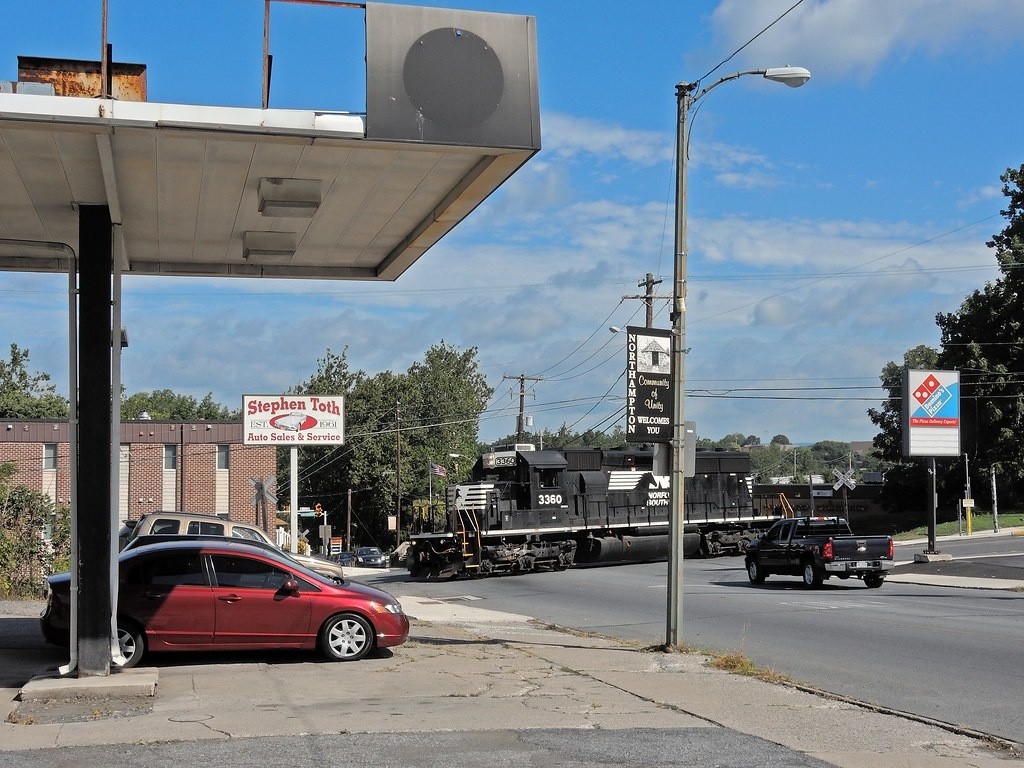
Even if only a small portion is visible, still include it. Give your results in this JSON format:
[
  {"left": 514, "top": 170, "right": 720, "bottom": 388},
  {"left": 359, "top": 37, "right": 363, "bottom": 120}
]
[
  {"left": 118, "top": 519, "right": 139, "bottom": 553},
  {"left": 130, "top": 511, "right": 345, "bottom": 586}
]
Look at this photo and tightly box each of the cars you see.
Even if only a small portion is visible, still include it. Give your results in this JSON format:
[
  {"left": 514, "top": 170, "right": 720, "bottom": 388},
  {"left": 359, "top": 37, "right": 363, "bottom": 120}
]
[
  {"left": 117, "top": 535, "right": 323, "bottom": 594},
  {"left": 337, "top": 551, "right": 357, "bottom": 567},
  {"left": 274, "top": 411, "right": 306, "bottom": 432},
  {"left": 331, "top": 554, "right": 338, "bottom": 562},
  {"left": 354, "top": 547, "right": 387, "bottom": 568},
  {"left": 37, "top": 540, "right": 410, "bottom": 661}
]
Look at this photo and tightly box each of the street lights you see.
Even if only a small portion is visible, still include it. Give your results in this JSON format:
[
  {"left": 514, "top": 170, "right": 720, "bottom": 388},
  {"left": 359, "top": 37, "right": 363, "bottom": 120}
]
[{"left": 663, "top": 63, "right": 813, "bottom": 654}]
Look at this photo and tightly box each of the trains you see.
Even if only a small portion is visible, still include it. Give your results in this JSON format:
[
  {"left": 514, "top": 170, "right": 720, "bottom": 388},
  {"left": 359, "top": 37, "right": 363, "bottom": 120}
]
[{"left": 402, "top": 443, "right": 928, "bottom": 583}]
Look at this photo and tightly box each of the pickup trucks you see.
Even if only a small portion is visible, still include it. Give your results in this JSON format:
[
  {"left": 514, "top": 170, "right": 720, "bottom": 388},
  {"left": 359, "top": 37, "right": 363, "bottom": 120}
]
[{"left": 745, "top": 517, "right": 895, "bottom": 591}]
